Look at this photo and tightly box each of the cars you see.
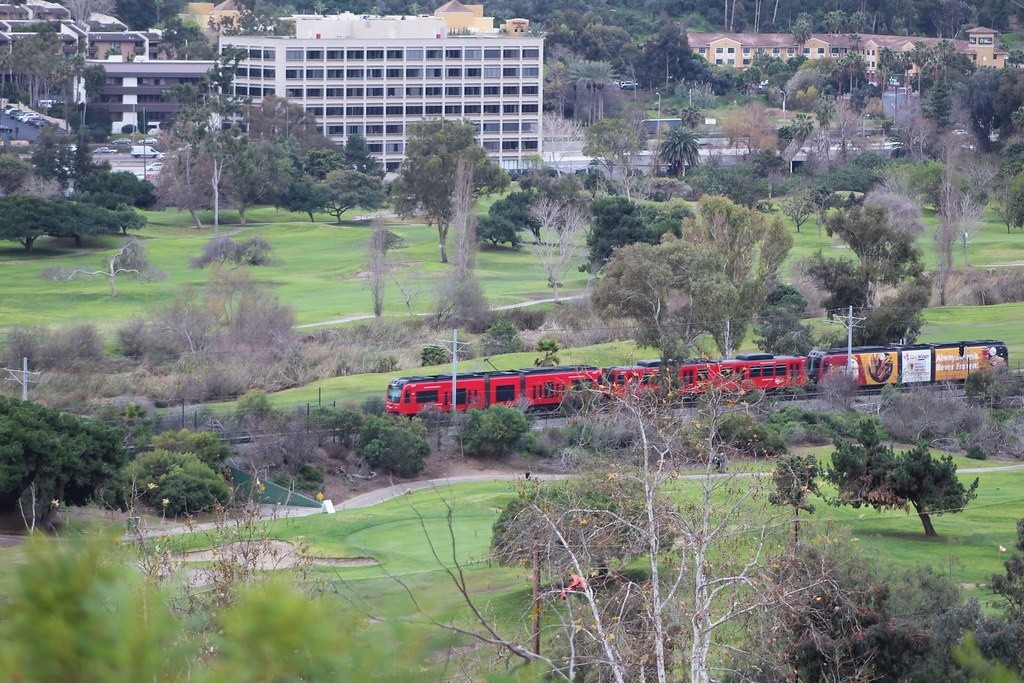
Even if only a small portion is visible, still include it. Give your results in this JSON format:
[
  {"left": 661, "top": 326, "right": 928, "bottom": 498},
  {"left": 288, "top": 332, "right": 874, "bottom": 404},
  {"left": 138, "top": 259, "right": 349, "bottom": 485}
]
[
  {"left": 112, "top": 138, "right": 131, "bottom": 144},
  {"left": 621, "top": 81, "right": 639, "bottom": 89},
  {"left": 138, "top": 137, "right": 158, "bottom": 144},
  {"left": 147, "top": 163, "right": 164, "bottom": 171},
  {"left": 4, "top": 100, "right": 56, "bottom": 127},
  {"left": 0, "top": 124, "right": 11, "bottom": 134}
]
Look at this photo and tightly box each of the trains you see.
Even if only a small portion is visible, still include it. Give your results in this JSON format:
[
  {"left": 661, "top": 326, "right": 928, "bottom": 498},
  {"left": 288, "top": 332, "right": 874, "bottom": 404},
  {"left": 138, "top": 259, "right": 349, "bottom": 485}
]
[{"left": 382, "top": 342, "right": 1010, "bottom": 422}]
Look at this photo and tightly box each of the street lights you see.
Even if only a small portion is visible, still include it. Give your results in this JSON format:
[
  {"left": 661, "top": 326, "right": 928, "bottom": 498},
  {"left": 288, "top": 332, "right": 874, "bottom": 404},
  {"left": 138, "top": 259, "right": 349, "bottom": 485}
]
[
  {"left": 653, "top": 92, "right": 662, "bottom": 150},
  {"left": 780, "top": 90, "right": 788, "bottom": 123}
]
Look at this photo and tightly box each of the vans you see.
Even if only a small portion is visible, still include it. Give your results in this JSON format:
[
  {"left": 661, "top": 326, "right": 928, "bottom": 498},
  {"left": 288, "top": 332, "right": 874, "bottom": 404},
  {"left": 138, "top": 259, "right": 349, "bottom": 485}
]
[{"left": 131, "top": 146, "right": 159, "bottom": 158}]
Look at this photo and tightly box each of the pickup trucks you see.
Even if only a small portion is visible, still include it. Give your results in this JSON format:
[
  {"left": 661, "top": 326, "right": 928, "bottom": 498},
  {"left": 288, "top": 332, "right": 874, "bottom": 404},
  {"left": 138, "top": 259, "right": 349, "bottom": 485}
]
[{"left": 93, "top": 147, "right": 117, "bottom": 154}]
